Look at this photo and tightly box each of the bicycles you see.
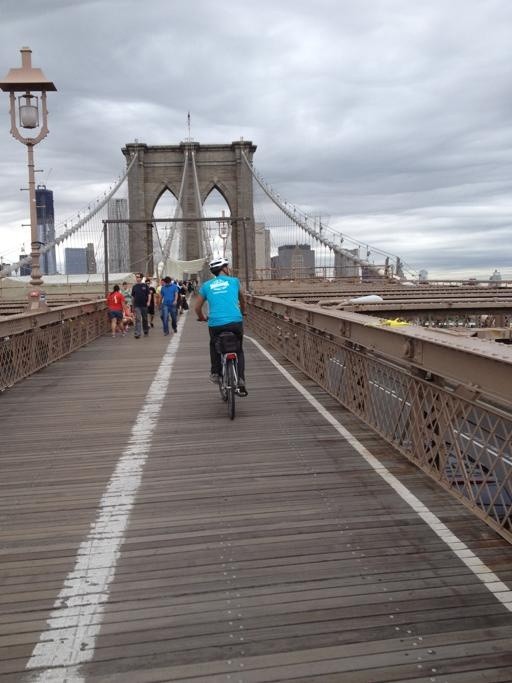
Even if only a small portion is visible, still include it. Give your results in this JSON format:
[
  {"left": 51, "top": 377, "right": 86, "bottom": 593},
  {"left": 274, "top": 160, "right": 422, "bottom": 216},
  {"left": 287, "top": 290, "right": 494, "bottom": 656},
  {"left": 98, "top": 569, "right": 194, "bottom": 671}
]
[{"left": 197, "top": 313, "right": 249, "bottom": 420}]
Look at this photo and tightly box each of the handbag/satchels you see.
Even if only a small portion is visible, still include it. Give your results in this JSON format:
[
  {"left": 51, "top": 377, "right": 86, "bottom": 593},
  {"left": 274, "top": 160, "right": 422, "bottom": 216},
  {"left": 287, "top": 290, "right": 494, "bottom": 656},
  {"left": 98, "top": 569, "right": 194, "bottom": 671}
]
[{"left": 182, "top": 296, "right": 188, "bottom": 311}]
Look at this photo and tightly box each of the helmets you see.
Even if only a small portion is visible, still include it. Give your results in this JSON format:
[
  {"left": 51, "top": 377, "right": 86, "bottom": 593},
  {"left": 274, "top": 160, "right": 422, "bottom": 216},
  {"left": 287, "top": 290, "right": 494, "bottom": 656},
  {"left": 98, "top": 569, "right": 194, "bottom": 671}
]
[{"left": 208, "top": 257, "right": 230, "bottom": 269}]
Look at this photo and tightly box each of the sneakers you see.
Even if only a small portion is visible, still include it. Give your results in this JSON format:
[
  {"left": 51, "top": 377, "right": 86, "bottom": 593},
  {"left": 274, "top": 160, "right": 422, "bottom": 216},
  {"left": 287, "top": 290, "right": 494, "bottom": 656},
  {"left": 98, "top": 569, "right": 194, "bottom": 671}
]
[
  {"left": 208, "top": 371, "right": 221, "bottom": 385},
  {"left": 237, "top": 375, "right": 248, "bottom": 398},
  {"left": 110, "top": 322, "right": 178, "bottom": 339}
]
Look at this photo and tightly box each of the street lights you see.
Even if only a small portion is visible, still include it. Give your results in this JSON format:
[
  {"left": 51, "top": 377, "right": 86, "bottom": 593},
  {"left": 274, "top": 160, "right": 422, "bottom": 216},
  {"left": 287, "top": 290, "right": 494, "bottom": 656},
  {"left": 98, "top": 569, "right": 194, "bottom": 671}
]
[
  {"left": 0, "top": 45, "right": 57, "bottom": 289},
  {"left": 217, "top": 209, "right": 233, "bottom": 258}
]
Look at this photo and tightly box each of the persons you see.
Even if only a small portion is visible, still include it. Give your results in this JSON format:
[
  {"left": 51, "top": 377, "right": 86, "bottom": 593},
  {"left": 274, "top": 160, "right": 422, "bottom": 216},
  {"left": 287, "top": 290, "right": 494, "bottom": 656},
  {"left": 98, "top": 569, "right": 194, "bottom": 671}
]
[
  {"left": 194, "top": 256, "right": 250, "bottom": 398},
  {"left": 105, "top": 272, "right": 199, "bottom": 338}
]
